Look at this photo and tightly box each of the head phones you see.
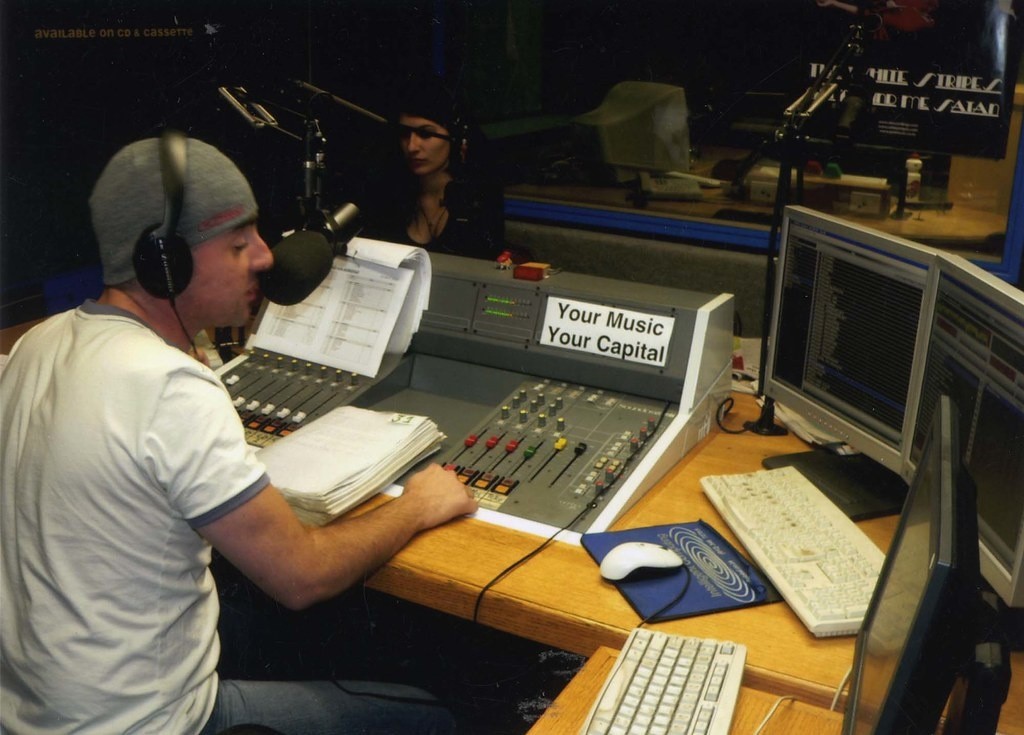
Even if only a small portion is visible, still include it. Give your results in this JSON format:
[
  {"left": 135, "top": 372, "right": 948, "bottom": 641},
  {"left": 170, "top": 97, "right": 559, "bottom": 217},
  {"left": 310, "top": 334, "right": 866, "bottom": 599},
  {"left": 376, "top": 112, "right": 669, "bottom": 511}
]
[
  {"left": 133, "top": 127, "right": 196, "bottom": 301},
  {"left": 446, "top": 85, "right": 477, "bottom": 165}
]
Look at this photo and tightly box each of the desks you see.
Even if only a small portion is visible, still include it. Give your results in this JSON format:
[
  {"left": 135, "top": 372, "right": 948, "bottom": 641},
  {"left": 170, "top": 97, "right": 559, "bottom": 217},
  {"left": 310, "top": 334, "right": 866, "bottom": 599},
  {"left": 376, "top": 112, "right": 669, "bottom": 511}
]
[{"left": 307, "top": 377, "right": 1024, "bottom": 735}]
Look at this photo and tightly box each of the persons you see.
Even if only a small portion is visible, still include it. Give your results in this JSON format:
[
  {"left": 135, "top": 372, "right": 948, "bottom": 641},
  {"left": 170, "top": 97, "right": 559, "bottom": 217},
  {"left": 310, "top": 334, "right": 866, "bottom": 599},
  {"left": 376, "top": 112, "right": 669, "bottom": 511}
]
[
  {"left": 0, "top": 138, "right": 476, "bottom": 735},
  {"left": 366, "top": 86, "right": 504, "bottom": 258}
]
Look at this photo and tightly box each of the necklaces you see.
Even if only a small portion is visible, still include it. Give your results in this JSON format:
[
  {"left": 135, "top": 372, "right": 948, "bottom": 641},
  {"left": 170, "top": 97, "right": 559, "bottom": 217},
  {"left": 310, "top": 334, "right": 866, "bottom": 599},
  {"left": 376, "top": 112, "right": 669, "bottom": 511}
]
[{"left": 419, "top": 204, "right": 446, "bottom": 238}]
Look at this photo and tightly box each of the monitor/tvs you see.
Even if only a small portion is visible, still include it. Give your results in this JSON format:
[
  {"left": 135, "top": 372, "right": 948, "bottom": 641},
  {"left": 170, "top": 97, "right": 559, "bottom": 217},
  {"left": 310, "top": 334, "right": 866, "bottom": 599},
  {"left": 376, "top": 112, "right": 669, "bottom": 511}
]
[
  {"left": 567, "top": 79, "right": 692, "bottom": 185},
  {"left": 761, "top": 204, "right": 1024, "bottom": 735}
]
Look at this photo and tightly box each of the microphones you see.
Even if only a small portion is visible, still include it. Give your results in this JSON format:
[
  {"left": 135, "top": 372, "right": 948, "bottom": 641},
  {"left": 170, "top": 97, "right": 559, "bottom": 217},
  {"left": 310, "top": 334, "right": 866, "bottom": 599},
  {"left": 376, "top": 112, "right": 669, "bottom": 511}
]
[
  {"left": 256, "top": 202, "right": 369, "bottom": 306},
  {"left": 835, "top": 93, "right": 867, "bottom": 138}
]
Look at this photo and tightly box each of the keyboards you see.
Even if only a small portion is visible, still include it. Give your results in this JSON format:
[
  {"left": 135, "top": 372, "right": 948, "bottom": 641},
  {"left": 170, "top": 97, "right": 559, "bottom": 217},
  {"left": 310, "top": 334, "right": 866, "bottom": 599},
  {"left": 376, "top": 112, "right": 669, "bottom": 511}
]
[
  {"left": 579, "top": 626, "right": 746, "bottom": 735},
  {"left": 700, "top": 463, "right": 886, "bottom": 637}
]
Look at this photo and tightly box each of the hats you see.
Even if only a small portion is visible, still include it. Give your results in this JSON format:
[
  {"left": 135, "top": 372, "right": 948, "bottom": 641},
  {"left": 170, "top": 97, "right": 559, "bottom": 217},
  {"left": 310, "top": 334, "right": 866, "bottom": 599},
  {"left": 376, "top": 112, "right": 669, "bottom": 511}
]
[{"left": 88, "top": 137, "right": 257, "bottom": 282}]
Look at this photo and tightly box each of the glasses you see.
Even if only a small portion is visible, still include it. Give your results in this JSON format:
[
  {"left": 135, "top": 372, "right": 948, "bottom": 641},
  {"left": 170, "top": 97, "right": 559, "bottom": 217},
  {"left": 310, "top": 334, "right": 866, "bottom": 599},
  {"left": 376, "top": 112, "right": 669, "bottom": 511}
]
[{"left": 399, "top": 125, "right": 454, "bottom": 144}]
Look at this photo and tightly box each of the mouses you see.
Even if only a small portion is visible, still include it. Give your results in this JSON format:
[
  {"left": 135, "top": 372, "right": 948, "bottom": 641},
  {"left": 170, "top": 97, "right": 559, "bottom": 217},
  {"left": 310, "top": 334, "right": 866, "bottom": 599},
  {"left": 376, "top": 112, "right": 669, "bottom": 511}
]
[{"left": 599, "top": 541, "right": 685, "bottom": 583}]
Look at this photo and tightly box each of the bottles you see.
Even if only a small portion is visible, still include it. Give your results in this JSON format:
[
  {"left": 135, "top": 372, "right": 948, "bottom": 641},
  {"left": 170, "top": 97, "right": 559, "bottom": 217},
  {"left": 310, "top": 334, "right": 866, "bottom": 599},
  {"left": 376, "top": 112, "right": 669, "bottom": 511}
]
[{"left": 906, "top": 153, "right": 922, "bottom": 202}]
